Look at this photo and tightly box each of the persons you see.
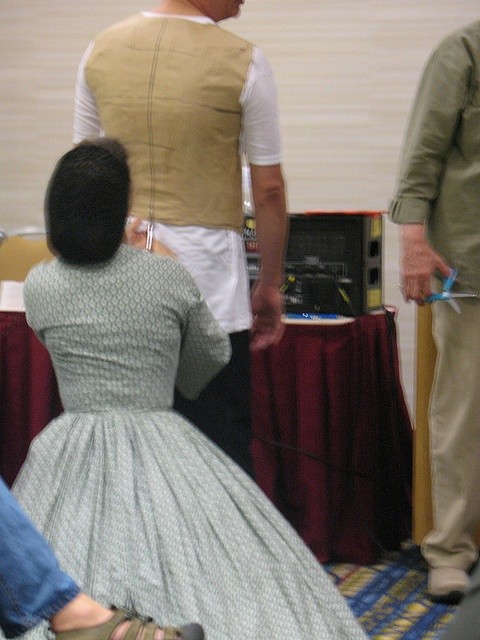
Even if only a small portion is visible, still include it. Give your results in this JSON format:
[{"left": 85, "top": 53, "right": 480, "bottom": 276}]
[
  {"left": 72, "top": 0, "right": 288, "bottom": 481},
  {"left": 386, "top": 18, "right": 480, "bottom": 606},
  {"left": 6, "top": 138, "right": 369, "bottom": 639},
  {"left": 1, "top": 474, "right": 206, "bottom": 640}
]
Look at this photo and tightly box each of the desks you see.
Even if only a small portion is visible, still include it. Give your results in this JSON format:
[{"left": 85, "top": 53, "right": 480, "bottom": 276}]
[{"left": 0, "top": 312, "right": 415, "bottom": 564}]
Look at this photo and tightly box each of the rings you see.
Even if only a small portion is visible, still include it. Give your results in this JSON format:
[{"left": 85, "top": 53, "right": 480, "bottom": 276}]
[{"left": 398, "top": 283, "right": 404, "bottom": 288}]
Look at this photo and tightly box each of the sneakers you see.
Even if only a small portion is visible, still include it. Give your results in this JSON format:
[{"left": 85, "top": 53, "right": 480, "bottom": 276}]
[
  {"left": 426, "top": 566, "right": 469, "bottom": 605},
  {"left": 43, "top": 602, "right": 204, "bottom": 640}
]
